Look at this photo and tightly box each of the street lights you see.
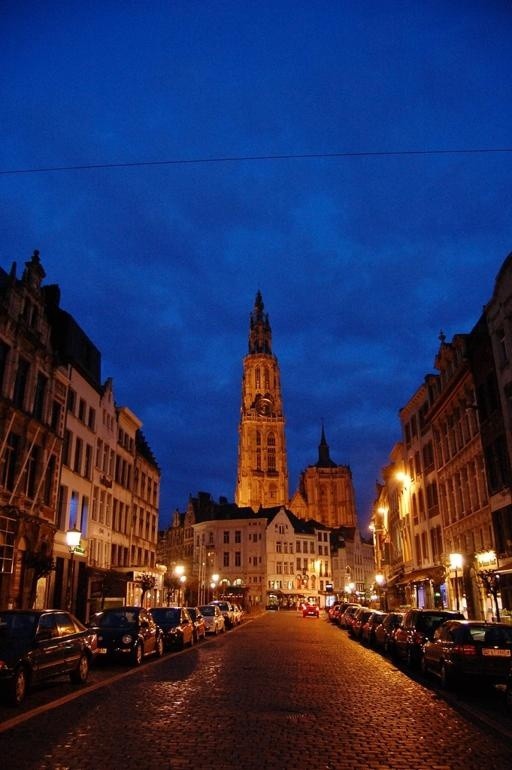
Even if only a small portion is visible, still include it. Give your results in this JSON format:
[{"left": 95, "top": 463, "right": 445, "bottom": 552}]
[
  {"left": 64, "top": 521, "right": 85, "bottom": 615},
  {"left": 447, "top": 550, "right": 465, "bottom": 613},
  {"left": 476, "top": 550, "right": 502, "bottom": 622},
  {"left": 177, "top": 576, "right": 186, "bottom": 607}
]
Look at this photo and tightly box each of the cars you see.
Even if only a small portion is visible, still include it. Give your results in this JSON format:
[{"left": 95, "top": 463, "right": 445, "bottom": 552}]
[
  {"left": 1, "top": 600, "right": 246, "bottom": 705},
  {"left": 299, "top": 600, "right": 511, "bottom": 687}
]
